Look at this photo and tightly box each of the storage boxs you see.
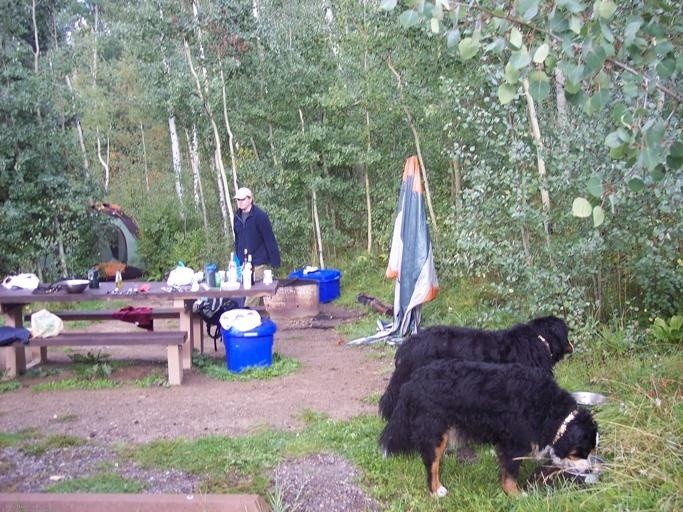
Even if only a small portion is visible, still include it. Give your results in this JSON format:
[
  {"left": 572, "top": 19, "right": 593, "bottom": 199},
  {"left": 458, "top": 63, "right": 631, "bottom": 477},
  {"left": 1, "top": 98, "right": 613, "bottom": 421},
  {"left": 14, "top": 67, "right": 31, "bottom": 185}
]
[{"left": 288, "top": 268, "right": 341, "bottom": 303}]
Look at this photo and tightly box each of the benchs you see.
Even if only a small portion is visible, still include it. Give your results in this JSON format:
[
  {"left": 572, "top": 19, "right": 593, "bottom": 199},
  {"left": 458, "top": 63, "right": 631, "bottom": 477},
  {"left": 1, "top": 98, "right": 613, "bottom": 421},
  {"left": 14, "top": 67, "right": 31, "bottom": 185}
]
[{"left": 6, "top": 304, "right": 203, "bottom": 386}]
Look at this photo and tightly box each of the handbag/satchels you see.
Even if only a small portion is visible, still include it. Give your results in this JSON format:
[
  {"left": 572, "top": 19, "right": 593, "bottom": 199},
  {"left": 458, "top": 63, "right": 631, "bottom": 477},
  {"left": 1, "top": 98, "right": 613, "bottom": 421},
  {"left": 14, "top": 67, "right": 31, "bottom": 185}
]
[{"left": 199, "top": 297, "right": 246, "bottom": 325}]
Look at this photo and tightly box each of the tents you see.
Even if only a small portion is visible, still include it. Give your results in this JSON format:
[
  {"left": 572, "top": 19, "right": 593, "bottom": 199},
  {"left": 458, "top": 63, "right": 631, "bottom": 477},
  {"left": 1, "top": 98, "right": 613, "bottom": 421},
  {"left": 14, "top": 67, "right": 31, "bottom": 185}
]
[{"left": 38, "top": 197, "right": 146, "bottom": 285}]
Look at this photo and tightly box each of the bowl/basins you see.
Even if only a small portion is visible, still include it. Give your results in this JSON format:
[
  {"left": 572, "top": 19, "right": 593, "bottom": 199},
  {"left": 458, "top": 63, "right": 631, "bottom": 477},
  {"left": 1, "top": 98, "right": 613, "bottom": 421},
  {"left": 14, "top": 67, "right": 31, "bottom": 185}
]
[
  {"left": 571, "top": 391, "right": 606, "bottom": 413},
  {"left": 57, "top": 278, "right": 91, "bottom": 294},
  {"left": 558, "top": 456, "right": 608, "bottom": 485}
]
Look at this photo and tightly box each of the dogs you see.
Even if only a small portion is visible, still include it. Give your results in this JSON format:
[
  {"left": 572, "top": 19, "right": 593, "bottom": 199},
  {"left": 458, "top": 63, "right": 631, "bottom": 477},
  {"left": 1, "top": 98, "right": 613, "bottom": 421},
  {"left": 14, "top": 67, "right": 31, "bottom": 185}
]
[
  {"left": 378, "top": 362, "right": 601, "bottom": 498},
  {"left": 377, "top": 315, "right": 576, "bottom": 464}
]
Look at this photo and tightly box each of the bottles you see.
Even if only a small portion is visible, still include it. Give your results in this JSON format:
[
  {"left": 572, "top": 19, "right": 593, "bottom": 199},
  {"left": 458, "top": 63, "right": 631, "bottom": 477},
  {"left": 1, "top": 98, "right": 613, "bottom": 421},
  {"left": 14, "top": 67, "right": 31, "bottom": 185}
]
[
  {"left": 206, "top": 247, "right": 273, "bottom": 292},
  {"left": 87, "top": 266, "right": 100, "bottom": 289},
  {"left": 115, "top": 271, "right": 122, "bottom": 292}
]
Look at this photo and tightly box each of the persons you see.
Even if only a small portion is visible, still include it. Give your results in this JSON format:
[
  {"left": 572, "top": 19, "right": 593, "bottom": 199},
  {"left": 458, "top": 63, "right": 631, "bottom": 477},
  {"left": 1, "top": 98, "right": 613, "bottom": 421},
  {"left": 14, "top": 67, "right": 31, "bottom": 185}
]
[{"left": 232, "top": 187, "right": 281, "bottom": 320}]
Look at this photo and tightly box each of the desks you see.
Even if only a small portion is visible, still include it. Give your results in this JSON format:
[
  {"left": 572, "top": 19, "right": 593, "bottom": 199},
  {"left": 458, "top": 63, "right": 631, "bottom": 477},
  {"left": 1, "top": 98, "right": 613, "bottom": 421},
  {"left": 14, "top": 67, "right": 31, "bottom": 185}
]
[{"left": 0, "top": 280, "right": 279, "bottom": 387}]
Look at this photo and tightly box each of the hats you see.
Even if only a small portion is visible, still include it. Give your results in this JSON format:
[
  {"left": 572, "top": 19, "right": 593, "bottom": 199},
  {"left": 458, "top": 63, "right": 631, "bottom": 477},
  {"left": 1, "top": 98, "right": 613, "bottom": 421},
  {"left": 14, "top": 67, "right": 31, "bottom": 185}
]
[{"left": 234, "top": 188, "right": 252, "bottom": 200}]
